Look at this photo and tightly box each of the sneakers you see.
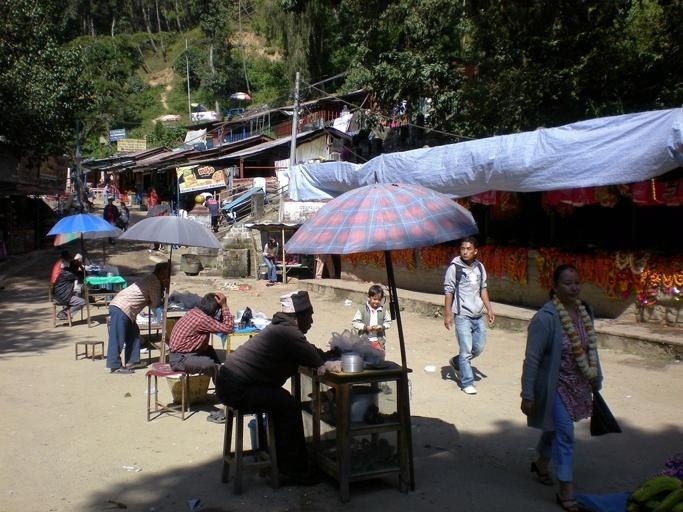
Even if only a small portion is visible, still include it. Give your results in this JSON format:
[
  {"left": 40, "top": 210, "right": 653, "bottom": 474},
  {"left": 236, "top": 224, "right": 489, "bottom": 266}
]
[
  {"left": 448, "top": 356, "right": 461, "bottom": 382},
  {"left": 458, "top": 385, "right": 477, "bottom": 395},
  {"left": 266, "top": 281, "right": 274, "bottom": 284},
  {"left": 381, "top": 385, "right": 393, "bottom": 394},
  {"left": 285, "top": 471, "right": 329, "bottom": 484},
  {"left": 56, "top": 311, "right": 72, "bottom": 320}
]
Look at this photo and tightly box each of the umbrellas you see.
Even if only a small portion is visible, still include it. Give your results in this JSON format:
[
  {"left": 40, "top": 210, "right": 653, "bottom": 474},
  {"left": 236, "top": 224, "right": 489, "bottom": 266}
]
[
  {"left": 53, "top": 232, "right": 114, "bottom": 271},
  {"left": 282, "top": 180, "right": 479, "bottom": 490},
  {"left": 230, "top": 92, "right": 251, "bottom": 109},
  {"left": 117, "top": 213, "right": 224, "bottom": 362},
  {"left": 46, "top": 213, "right": 119, "bottom": 328},
  {"left": 83, "top": 227, "right": 124, "bottom": 263}
]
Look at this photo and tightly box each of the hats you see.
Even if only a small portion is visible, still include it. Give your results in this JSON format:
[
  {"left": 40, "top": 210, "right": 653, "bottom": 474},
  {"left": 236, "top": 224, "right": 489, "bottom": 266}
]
[{"left": 278, "top": 290, "right": 313, "bottom": 313}]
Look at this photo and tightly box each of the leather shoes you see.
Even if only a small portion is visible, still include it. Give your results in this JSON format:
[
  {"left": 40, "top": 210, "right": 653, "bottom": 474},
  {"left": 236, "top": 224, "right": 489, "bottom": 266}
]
[
  {"left": 110, "top": 365, "right": 134, "bottom": 373},
  {"left": 125, "top": 359, "right": 148, "bottom": 369}
]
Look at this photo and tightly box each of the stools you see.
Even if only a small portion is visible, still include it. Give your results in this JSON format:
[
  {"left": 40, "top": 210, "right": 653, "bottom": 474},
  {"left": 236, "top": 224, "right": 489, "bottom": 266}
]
[
  {"left": 52, "top": 301, "right": 83, "bottom": 327},
  {"left": 217, "top": 403, "right": 279, "bottom": 495},
  {"left": 146, "top": 362, "right": 187, "bottom": 421},
  {"left": 76, "top": 340, "right": 104, "bottom": 361}
]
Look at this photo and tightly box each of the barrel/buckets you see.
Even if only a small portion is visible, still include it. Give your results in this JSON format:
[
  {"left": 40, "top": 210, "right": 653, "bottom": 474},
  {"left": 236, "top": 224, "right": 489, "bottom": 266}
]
[{"left": 249, "top": 419, "right": 268, "bottom": 462}]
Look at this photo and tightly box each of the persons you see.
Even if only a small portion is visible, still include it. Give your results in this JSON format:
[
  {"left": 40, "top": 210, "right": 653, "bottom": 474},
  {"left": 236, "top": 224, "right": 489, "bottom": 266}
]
[
  {"left": 102, "top": 179, "right": 129, "bottom": 245},
  {"left": 106, "top": 263, "right": 169, "bottom": 373},
  {"left": 53, "top": 259, "right": 87, "bottom": 319},
  {"left": 521, "top": 264, "right": 603, "bottom": 512},
  {"left": 354, "top": 286, "right": 393, "bottom": 396},
  {"left": 170, "top": 292, "right": 234, "bottom": 385},
  {"left": 149, "top": 188, "right": 158, "bottom": 209},
  {"left": 215, "top": 289, "right": 343, "bottom": 487},
  {"left": 314, "top": 254, "right": 335, "bottom": 280},
  {"left": 263, "top": 234, "right": 280, "bottom": 283},
  {"left": 204, "top": 195, "right": 221, "bottom": 233},
  {"left": 70, "top": 253, "right": 83, "bottom": 275},
  {"left": 444, "top": 238, "right": 495, "bottom": 394},
  {"left": 50, "top": 251, "right": 65, "bottom": 284}
]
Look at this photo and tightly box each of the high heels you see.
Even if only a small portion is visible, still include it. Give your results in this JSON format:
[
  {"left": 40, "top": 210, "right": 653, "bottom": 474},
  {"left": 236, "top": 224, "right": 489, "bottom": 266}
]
[
  {"left": 529, "top": 461, "right": 554, "bottom": 486},
  {"left": 553, "top": 493, "right": 580, "bottom": 511}
]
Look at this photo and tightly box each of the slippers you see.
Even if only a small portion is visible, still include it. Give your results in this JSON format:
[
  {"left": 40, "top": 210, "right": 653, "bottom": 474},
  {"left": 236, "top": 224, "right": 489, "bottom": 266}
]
[{"left": 206, "top": 412, "right": 226, "bottom": 424}]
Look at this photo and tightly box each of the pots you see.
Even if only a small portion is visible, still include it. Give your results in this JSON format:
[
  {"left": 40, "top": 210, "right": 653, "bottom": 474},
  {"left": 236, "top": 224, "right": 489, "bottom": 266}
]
[{"left": 329, "top": 386, "right": 382, "bottom": 423}]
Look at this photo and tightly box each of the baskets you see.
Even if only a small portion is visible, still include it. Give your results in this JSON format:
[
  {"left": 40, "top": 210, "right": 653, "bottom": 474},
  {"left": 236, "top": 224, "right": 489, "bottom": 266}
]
[{"left": 166, "top": 374, "right": 211, "bottom": 403}]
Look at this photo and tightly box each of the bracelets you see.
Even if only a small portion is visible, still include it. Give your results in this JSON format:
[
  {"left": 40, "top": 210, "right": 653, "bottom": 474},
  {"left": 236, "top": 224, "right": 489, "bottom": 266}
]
[{"left": 371, "top": 325, "right": 374, "bottom": 331}]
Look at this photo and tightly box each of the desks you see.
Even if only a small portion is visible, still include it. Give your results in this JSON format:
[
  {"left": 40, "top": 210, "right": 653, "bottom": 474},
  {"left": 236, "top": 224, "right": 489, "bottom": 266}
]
[
  {"left": 211, "top": 326, "right": 261, "bottom": 361},
  {"left": 84, "top": 276, "right": 127, "bottom": 328},
  {"left": 291, "top": 359, "right": 416, "bottom": 503},
  {"left": 259, "top": 264, "right": 314, "bottom": 283},
  {"left": 165, "top": 311, "right": 187, "bottom": 345}
]
